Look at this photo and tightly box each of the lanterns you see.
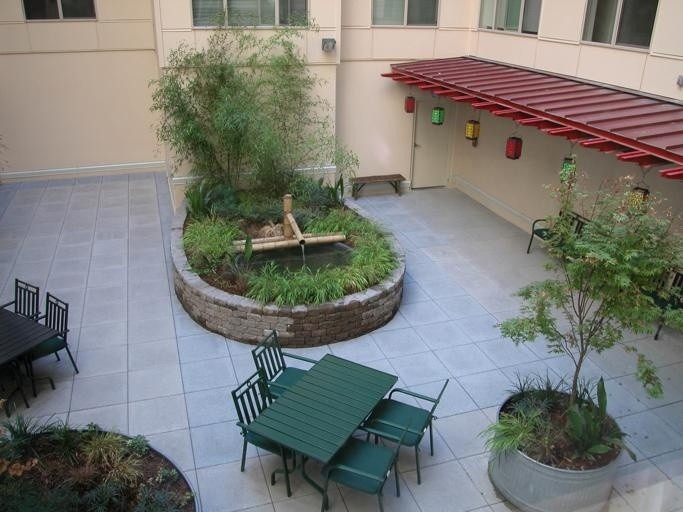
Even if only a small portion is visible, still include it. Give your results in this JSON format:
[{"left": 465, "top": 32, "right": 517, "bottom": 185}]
[
  {"left": 504, "top": 137, "right": 523, "bottom": 160},
  {"left": 464, "top": 119, "right": 480, "bottom": 141},
  {"left": 431, "top": 107, "right": 444, "bottom": 126},
  {"left": 559, "top": 157, "right": 576, "bottom": 183},
  {"left": 631, "top": 185, "right": 651, "bottom": 215},
  {"left": 403, "top": 95, "right": 415, "bottom": 114}
]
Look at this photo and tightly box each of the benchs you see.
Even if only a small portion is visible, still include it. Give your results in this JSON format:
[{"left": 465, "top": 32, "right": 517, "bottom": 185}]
[
  {"left": 526, "top": 206, "right": 682, "bottom": 341},
  {"left": 347, "top": 174, "right": 405, "bottom": 200}
]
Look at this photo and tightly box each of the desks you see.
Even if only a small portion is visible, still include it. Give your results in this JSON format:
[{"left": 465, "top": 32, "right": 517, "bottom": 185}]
[{"left": 248, "top": 352, "right": 399, "bottom": 508}]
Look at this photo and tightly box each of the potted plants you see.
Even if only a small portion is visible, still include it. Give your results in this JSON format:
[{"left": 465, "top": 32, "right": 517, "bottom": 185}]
[{"left": 471, "top": 154, "right": 683, "bottom": 511}]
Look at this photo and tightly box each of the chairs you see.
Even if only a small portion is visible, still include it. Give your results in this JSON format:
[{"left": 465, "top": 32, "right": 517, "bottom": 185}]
[
  {"left": 232, "top": 368, "right": 298, "bottom": 498},
  {"left": 320, "top": 424, "right": 409, "bottom": 511},
  {"left": 252, "top": 329, "right": 318, "bottom": 411},
  {"left": 357, "top": 379, "right": 449, "bottom": 485},
  {"left": 0, "top": 277, "right": 79, "bottom": 398}
]
